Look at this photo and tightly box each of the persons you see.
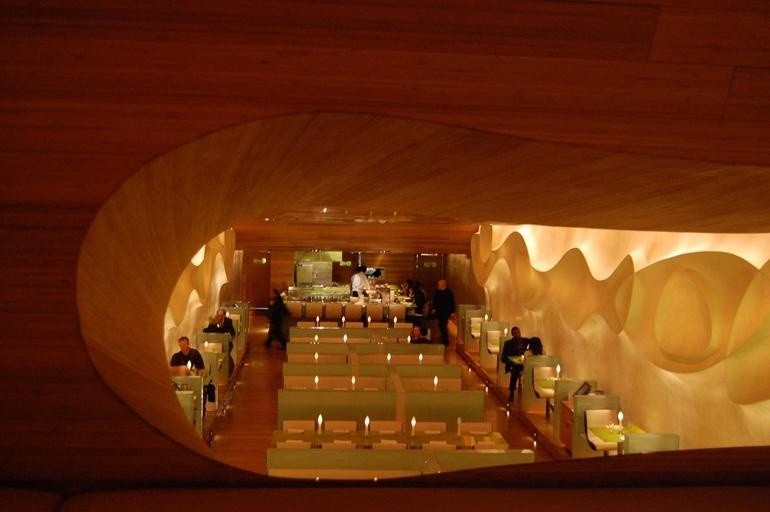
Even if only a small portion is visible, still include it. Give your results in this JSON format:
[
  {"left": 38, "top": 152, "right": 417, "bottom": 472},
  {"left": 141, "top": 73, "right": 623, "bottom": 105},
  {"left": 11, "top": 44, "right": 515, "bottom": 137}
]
[
  {"left": 351, "top": 264, "right": 370, "bottom": 298},
  {"left": 202, "top": 311, "right": 223, "bottom": 333},
  {"left": 169, "top": 336, "right": 205, "bottom": 369},
  {"left": 261, "top": 288, "right": 287, "bottom": 350},
  {"left": 500, "top": 327, "right": 533, "bottom": 394},
  {"left": 216, "top": 307, "right": 235, "bottom": 336},
  {"left": 523, "top": 337, "right": 546, "bottom": 365},
  {"left": 401, "top": 278, "right": 431, "bottom": 343},
  {"left": 430, "top": 279, "right": 455, "bottom": 342}
]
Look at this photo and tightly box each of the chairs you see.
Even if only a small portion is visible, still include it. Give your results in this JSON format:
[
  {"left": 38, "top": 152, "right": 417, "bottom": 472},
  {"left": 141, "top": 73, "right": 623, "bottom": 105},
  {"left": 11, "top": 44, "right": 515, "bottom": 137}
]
[
  {"left": 617, "top": 438, "right": 627, "bottom": 458},
  {"left": 265, "top": 302, "right": 534, "bottom": 481},
  {"left": 583, "top": 407, "right": 625, "bottom": 456},
  {"left": 172, "top": 305, "right": 251, "bottom": 441},
  {"left": 533, "top": 365, "right": 561, "bottom": 422}
]
